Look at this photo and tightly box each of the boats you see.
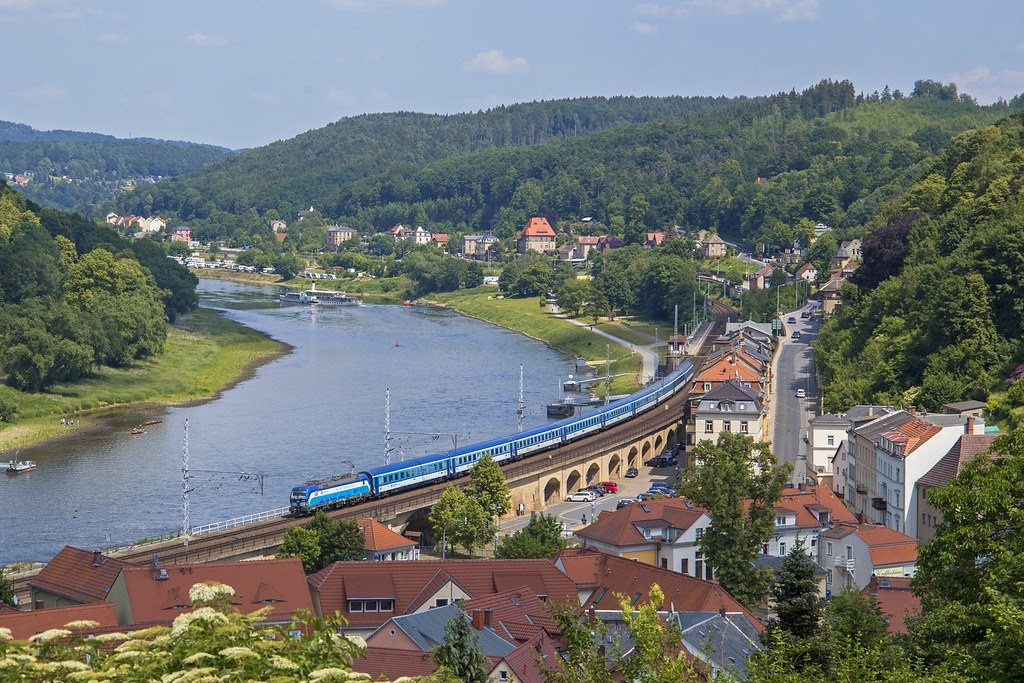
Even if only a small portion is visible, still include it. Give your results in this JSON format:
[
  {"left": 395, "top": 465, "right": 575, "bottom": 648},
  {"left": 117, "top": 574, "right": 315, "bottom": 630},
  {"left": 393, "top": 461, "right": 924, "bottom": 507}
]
[
  {"left": 143, "top": 418, "right": 162, "bottom": 426},
  {"left": 563, "top": 379, "right": 578, "bottom": 391},
  {"left": 546, "top": 395, "right": 576, "bottom": 416},
  {"left": 0, "top": 451, "right": 37, "bottom": 472},
  {"left": 131, "top": 427, "right": 146, "bottom": 434},
  {"left": 278, "top": 279, "right": 362, "bottom": 306}
]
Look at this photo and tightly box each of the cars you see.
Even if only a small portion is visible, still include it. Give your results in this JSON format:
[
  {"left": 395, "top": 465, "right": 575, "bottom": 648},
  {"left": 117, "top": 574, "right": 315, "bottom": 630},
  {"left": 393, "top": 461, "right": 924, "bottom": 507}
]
[
  {"left": 563, "top": 439, "right": 689, "bottom": 510},
  {"left": 626, "top": 466, "right": 638, "bottom": 477},
  {"left": 791, "top": 331, "right": 801, "bottom": 338},
  {"left": 786, "top": 316, "right": 796, "bottom": 323},
  {"left": 801, "top": 303, "right": 819, "bottom": 319},
  {"left": 796, "top": 388, "right": 806, "bottom": 398}
]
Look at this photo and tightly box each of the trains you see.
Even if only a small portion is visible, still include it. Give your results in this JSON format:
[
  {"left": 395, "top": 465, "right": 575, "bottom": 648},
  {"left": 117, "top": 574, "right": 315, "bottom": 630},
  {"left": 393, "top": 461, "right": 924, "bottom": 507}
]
[{"left": 289, "top": 359, "right": 695, "bottom": 520}]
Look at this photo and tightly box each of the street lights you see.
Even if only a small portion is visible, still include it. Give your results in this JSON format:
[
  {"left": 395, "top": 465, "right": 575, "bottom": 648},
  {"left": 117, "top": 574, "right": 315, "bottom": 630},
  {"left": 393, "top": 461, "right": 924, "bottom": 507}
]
[
  {"left": 795, "top": 279, "right": 804, "bottom": 308},
  {"left": 775, "top": 284, "right": 786, "bottom": 336}
]
